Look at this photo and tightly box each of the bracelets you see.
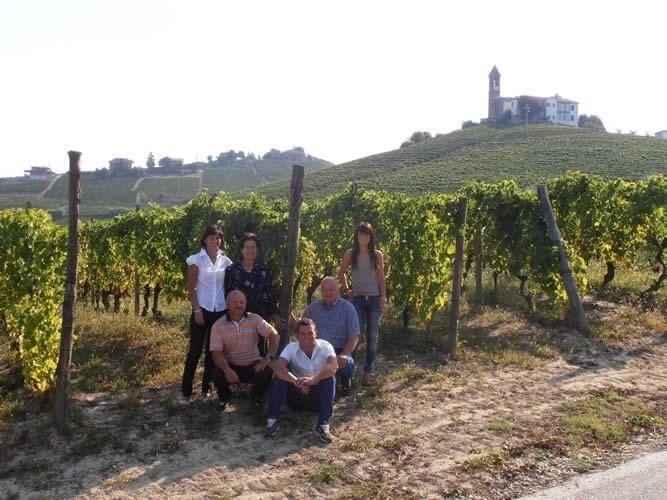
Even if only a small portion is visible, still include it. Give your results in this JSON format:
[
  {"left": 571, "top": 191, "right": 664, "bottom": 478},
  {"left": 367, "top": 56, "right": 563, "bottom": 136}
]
[{"left": 194, "top": 309, "right": 202, "bottom": 313}]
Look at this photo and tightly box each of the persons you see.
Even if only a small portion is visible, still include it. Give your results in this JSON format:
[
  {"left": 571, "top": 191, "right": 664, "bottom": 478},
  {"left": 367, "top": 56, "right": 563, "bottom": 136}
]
[
  {"left": 339, "top": 222, "right": 385, "bottom": 385},
  {"left": 209, "top": 289, "right": 281, "bottom": 413},
  {"left": 223, "top": 232, "right": 286, "bottom": 357},
  {"left": 290, "top": 276, "right": 360, "bottom": 397},
  {"left": 264, "top": 319, "right": 338, "bottom": 443},
  {"left": 179, "top": 225, "right": 233, "bottom": 406}
]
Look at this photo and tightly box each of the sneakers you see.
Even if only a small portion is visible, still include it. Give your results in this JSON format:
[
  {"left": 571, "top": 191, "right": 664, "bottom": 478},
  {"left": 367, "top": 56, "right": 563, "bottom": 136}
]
[
  {"left": 342, "top": 378, "right": 356, "bottom": 395},
  {"left": 265, "top": 419, "right": 282, "bottom": 437},
  {"left": 216, "top": 399, "right": 231, "bottom": 411},
  {"left": 362, "top": 370, "right": 373, "bottom": 386},
  {"left": 313, "top": 422, "right": 335, "bottom": 444},
  {"left": 198, "top": 392, "right": 209, "bottom": 404},
  {"left": 179, "top": 396, "right": 190, "bottom": 410},
  {"left": 253, "top": 401, "right": 263, "bottom": 407}
]
